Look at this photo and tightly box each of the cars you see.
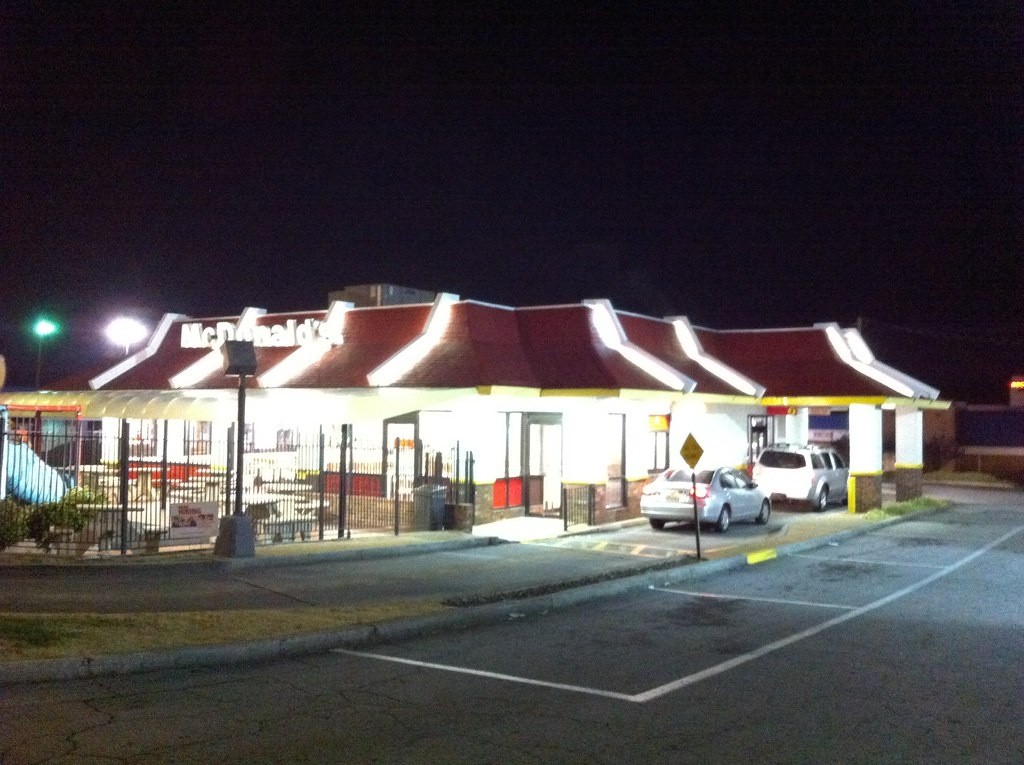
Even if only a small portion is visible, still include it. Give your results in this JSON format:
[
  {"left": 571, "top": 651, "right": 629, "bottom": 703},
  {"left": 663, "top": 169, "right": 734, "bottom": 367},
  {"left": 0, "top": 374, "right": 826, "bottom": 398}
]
[
  {"left": 750, "top": 442, "right": 849, "bottom": 511},
  {"left": 639, "top": 463, "right": 770, "bottom": 531}
]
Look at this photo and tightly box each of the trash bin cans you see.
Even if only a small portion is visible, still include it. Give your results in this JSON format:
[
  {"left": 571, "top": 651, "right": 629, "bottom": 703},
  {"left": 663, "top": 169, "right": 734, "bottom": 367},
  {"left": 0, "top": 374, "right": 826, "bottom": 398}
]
[{"left": 413, "top": 485, "right": 447, "bottom": 531}]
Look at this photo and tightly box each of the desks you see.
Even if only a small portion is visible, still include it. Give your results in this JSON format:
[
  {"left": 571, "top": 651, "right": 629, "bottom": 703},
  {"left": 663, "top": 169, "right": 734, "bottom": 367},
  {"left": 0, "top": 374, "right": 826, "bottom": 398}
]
[{"left": 56, "top": 461, "right": 329, "bottom": 545}]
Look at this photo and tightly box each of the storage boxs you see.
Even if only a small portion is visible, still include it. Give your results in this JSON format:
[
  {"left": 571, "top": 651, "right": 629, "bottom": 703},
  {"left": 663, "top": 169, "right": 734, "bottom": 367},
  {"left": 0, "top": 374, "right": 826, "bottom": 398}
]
[{"left": 413, "top": 484, "right": 447, "bottom": 532}]
